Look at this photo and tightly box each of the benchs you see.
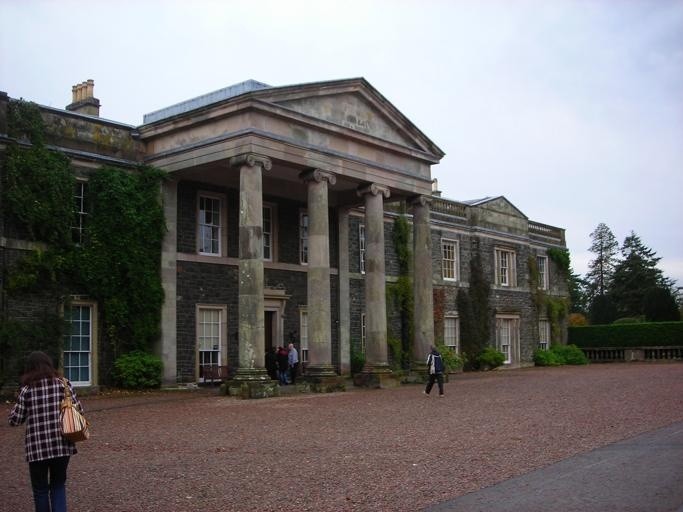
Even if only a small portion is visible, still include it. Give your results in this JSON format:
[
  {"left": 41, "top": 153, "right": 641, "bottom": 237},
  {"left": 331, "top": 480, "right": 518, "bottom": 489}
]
[{"left": 202, "top": 365, "right": 230, "bottom": 388}]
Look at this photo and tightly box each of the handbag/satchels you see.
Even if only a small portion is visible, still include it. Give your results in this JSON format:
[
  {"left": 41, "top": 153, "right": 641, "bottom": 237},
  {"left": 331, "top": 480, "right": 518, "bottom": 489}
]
[{"left": 59, "top": 375, "right": 90, "bottom": 443}]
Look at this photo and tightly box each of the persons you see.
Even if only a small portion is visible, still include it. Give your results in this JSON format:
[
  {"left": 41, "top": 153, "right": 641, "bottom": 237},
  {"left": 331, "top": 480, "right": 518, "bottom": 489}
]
[
  {"left": 286, "top": 344, "right": 298, "bottom": 385},
  {"left": 8, "top": 349, "right": 83, "bottom": 511},
  {"left": 264, "top": 346, "right": 277, "bottom": 379},
  {"left": 275, "top": 346, "right": 290, "bottom": 385},
  {"left": 421, "top": 344, "right": 445, "bottom": 398}
]
